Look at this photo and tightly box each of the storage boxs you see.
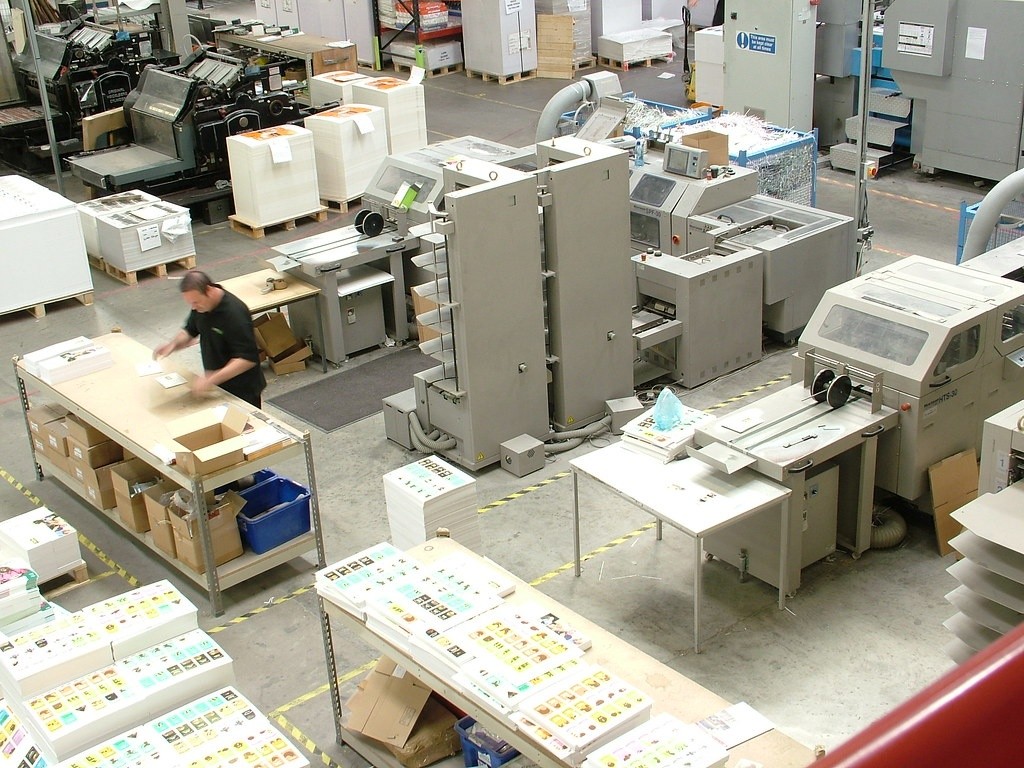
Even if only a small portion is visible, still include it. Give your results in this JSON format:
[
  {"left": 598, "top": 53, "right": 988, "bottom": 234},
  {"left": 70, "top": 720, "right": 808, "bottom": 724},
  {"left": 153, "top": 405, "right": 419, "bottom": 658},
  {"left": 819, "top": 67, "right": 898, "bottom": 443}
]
[
  {"left": 695, "top": 25, "right": 725, "bottom": 106},
  {"left": 453, "top": 714, "right": 522, "bottom": 768},
  {"left": 410, "top": 287, "right": 445, "bottom": 343},
  {"left": 28, "top": 400, "right": 314, "bottom": 574},
  {"left": 81, "top": 107, "right": 127, "bottom": 200},
  {"left": 682, "top": 129, "right": 730, "bottom": 168},
  {"left": 252, "top": 312, "right": 313, "bottom": 375},
  {"left": 344, "top": 657, "right": 463, "bottom": 768}
]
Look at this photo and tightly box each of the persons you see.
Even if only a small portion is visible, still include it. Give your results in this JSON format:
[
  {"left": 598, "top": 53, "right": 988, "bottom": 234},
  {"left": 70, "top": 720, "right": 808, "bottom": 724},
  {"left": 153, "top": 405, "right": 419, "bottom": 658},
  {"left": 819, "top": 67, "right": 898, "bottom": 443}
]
[
  {"left": 689, "top": 0, "right": 725, "bottom": 26},
  {"left": 153, "top": 270, "right": 267, "bottom": 409}
]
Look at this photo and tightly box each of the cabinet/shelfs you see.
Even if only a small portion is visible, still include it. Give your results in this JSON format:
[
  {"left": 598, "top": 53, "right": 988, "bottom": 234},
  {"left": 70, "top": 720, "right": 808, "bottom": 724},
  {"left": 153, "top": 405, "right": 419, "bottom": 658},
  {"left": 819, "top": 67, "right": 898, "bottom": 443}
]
[{"left": 370, "top": 0, "right": 464, "bottom": 82}]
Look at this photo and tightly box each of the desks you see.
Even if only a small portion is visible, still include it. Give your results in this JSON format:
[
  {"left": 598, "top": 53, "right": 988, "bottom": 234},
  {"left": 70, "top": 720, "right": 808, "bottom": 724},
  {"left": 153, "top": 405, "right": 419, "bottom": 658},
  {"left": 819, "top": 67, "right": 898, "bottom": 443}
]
[
  {"left": 568, "top": 440, "right": 793, "bottom": 653},
  {"left": 215, "top": 268, "right": 328, "bottom": 373},
  {"left": 318, "top": 529, "right": 823, "bottom": 768},
  {"left": 214, "top": 29, "right": 359, "bottom": 79},
  {"left": 12, "top": 326, "right": 326, "bottom": 616}
]
